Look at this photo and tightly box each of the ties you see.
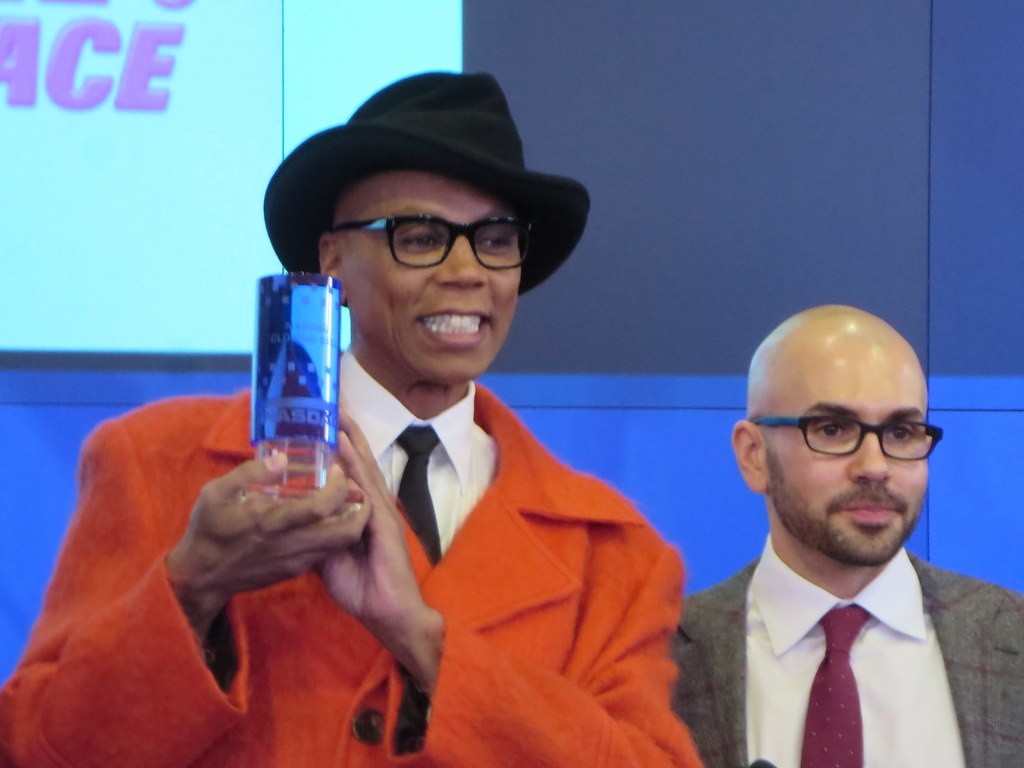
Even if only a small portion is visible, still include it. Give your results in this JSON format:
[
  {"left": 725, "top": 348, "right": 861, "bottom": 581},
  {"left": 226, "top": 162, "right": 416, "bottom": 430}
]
[
  {"left": 800, "top": 601, "right": 869, "bottom": 766},
  {"left": 393, "top": 421, "right": 441, "bottom": 566}
]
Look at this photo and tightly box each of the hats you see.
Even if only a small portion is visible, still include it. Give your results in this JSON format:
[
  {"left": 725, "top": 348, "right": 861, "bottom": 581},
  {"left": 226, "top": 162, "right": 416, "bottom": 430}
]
[{"left": 262, "top": 71, "right": 593, "bottom": 295}]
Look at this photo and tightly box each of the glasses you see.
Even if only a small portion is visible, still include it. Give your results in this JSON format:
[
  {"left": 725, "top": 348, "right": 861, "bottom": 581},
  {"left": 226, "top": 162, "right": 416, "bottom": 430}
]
[
  {"left": 749, "top": 413, "right": 944, "bottom": 462},
  {"left": 326, "top": 215, "right": 537, "bottom": 269}
]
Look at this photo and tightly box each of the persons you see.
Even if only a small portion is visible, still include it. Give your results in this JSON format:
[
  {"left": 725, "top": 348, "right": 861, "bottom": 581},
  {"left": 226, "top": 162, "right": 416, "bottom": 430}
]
[
  {"left": 668, "top": 301, "right": 1024, "bottom": 767},
  {"left": 0, "top": 72, "right": 706, "bottom": 767}
]
